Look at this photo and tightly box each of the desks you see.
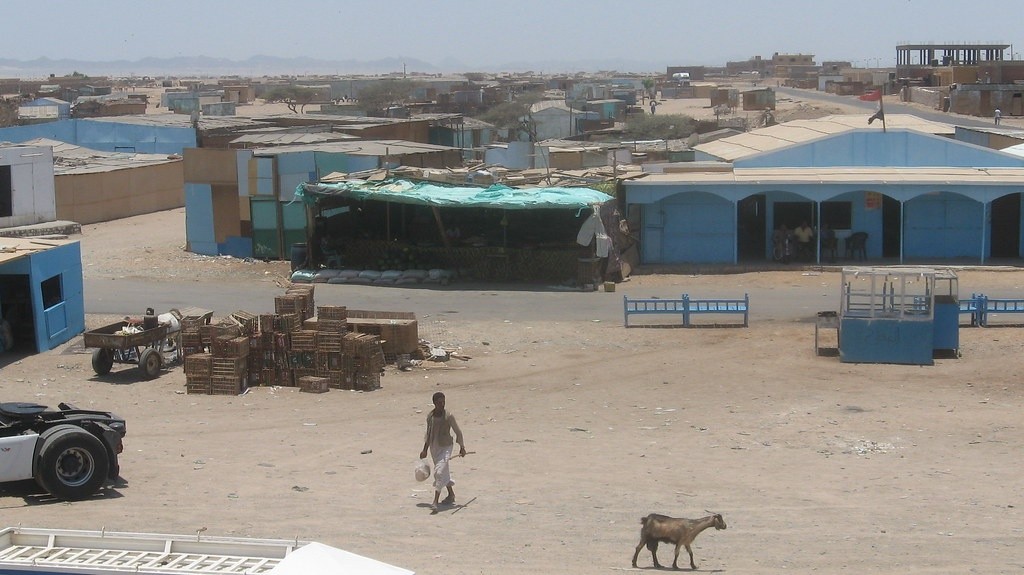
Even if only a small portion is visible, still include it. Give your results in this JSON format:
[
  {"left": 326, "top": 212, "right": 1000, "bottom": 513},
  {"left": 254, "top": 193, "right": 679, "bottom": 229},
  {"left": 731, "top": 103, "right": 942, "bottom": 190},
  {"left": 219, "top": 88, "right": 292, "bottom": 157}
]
[
  {"left": 815, "top": 310, "right": 841, "bottom": 356},
  {"left": 576, "top": 257, "right": 601, "bottom": 288},
  {"left": 483, "top": 252, "right": 514, "bottom": 283}
]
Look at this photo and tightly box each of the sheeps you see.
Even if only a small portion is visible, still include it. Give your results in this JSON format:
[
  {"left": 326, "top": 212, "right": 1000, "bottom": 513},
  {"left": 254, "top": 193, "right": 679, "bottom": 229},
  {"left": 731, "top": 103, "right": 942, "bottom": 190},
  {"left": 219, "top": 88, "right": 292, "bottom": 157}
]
[{"left": 633, "top": 511, "right": 727, "bottom": 570}]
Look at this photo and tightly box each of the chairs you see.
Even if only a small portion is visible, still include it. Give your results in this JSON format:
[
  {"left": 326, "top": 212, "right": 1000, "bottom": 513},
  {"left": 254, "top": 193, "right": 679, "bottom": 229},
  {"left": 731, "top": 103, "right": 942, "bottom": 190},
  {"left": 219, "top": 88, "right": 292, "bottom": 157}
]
[
  {"left": 844, "top": 232, "right": 868, "bottom": 262},
  {"left": 793, "top": 227, "right": 816, "bottom": 262},
  {"left": 821, "top": 238, "right": 838, "bottom": 263}
]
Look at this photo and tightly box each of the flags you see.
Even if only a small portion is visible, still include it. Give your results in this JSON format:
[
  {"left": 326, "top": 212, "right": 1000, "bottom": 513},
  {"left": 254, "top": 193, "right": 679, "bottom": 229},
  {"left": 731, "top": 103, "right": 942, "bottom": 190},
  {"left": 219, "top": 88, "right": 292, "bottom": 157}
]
[
  {"left": 868, "top": 110, "right": 884, "bottom": 124},
  {"left": 858, "top": 88, "right": 880, "bottom": 101}
]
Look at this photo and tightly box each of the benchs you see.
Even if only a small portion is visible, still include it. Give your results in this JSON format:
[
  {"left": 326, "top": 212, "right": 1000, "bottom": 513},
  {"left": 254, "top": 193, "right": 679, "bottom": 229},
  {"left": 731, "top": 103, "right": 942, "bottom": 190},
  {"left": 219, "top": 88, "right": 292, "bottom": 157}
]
[
  {"left": 686, "top": 293, "right": 749, "bottom": 328},
  {"left": 912, "top": 293, "right": 981, "bottom": 327},
  {"left": 622, "top": 294, "right": 685, "bottom": 328},
  {"left": 980, "top": 294, "right": 1024, "bottom": 327}
]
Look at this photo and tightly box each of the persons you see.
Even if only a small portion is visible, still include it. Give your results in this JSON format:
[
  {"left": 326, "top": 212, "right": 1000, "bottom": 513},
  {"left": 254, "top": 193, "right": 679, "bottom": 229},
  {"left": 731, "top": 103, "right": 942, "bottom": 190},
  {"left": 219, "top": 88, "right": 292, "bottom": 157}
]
[
  {"left": 420, "top": 392, "right": 466, "bottom": 512},
  {"left": 772, "top": 224, "right": 796, "bottom": 263},
  {"left": 446, "top": 223, "right": 461, "bottom": 238},
  {"left": 793, "top": 220, "right": 815, "bottom": 263},
  {"left": 994, "top": 107, "right": 1001, "bottom": 125},
  {"left": 821, "top": 223, "right": 831, "bottom": 241}
]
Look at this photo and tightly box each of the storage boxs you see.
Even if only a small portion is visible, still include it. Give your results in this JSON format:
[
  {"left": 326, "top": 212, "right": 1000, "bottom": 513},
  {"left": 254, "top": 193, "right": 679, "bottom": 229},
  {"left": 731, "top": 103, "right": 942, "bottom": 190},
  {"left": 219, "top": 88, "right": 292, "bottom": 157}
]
[{"left": 180, "top": 283, "right": 387, "bottom": 395}]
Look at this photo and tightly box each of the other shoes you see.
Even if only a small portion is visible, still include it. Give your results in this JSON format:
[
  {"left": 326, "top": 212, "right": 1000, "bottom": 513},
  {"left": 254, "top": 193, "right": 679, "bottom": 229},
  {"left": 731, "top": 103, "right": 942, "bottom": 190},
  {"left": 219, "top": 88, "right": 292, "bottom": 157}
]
[
  {"left": 432, "top": 502, "right": 439, "bottom": 510},
  {"left": 442, "top": 496, "right": 455, "bottom": 503}
]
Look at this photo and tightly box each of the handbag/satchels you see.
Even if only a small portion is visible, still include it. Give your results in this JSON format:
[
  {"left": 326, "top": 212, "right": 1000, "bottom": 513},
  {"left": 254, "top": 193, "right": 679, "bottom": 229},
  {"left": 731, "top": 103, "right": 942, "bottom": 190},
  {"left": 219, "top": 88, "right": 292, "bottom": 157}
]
[{"left": 415, "top": 457, "right": 431, "bottom": 482}]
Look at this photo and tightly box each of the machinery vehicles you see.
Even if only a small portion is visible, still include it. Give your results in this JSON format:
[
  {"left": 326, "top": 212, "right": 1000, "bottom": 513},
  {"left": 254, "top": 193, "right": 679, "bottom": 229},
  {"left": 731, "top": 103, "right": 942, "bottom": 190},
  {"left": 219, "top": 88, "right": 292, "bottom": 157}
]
[{"left": 0, "top": 403, "right": 125, "bottom": 502}]
